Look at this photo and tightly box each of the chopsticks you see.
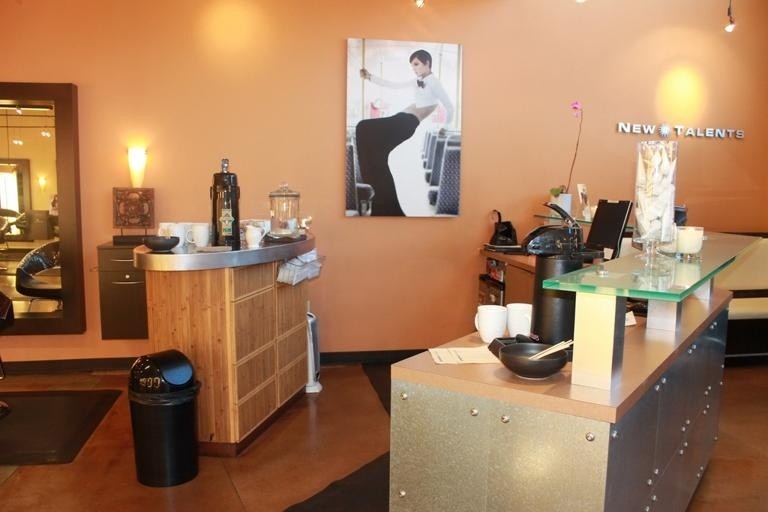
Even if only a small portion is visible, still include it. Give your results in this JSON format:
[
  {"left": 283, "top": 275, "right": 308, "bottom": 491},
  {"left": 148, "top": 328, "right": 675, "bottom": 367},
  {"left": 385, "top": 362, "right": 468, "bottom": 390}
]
[{"left": 529, "top": 338, "right": 574, "bottom": 361}]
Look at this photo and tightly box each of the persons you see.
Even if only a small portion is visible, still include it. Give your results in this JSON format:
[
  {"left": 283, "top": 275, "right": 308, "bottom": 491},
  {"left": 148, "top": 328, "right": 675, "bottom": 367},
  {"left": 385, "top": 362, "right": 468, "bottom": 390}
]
[{"left": 356, "top": 49, "right": 455, "bottom": 217}]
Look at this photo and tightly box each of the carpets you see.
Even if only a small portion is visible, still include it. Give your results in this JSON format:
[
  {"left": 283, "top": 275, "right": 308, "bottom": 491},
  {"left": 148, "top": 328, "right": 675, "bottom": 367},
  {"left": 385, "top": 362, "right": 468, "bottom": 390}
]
[
  {"left": 284, "top": 451, "right": 391, "bottom": 511},
  {"left": 360, "top": 356, "right": 412, "bottom": 413},
  {"left": 0, "top": 387, "right": 124, "bottom": 468}
]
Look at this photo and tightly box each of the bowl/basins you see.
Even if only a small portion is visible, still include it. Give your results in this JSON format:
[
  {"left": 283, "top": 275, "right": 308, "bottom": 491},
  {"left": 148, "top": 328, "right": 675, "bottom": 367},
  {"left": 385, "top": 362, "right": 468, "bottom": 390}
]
[
  {"left": 498, "top": 342, "right": 568, "bottom": 381},
  {"left": 142, "top": 235, "right": 179, "bottom": 252}
]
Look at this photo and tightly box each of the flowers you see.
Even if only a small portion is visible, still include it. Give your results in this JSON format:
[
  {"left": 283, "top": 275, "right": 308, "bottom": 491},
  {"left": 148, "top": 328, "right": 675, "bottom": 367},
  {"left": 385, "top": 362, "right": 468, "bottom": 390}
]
[{"left": 549, "top": 100, "right": 584, "bottom": 197}]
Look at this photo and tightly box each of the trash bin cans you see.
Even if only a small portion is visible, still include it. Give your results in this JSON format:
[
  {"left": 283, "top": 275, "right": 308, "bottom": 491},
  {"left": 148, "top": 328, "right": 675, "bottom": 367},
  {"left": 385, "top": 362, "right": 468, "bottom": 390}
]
[{"left": 127, "top": 348, "right": 203, "bottom": 488}]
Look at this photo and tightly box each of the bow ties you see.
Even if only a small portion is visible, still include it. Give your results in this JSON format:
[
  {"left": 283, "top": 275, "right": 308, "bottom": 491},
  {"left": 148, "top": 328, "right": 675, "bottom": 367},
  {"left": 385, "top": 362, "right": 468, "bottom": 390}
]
[{"left": 417, "top": 80, "right": 424, "bottom": 87}]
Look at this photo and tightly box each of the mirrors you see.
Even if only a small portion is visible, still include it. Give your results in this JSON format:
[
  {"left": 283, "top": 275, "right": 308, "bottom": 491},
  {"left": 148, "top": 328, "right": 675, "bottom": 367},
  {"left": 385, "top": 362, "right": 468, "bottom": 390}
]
[
  {"left": 1, "top": 158, "right": 34, "bottom": 242},
  {"left": 1, "top": 83, "right": 86, "bottom": 336}
]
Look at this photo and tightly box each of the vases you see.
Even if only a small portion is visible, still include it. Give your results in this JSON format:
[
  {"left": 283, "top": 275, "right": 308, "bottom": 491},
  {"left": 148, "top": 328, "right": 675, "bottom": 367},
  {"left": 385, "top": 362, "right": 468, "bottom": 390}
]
[{"left": 543, "top": 194, "right": 571, "bottom": 228}]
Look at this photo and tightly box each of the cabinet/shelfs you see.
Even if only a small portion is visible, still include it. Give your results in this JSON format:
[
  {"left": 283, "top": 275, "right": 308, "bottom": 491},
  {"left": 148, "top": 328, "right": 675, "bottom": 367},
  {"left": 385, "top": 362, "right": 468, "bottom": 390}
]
[
  {"left": 97, "top": 241, "right": 148, "bottom": 339},
  {"left": 29, "top": 209, "right": 49, "bottom": 239},
  {"left": 134, "top": 227, "right": 316, "bottom": 459},
  {"left": 386, "top": 226, "right": 763, "bottom": 512},
  {"left": 477, "top": 211, "right": 640, "bottom": 309}
]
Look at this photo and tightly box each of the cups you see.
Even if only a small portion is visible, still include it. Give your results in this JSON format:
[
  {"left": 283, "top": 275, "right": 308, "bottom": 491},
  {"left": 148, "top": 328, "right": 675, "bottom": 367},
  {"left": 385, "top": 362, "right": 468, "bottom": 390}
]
[
  {"left": 186, "top": 224, "right": 209, "bottom": 248},
  {"left": 246, "top": 225, "right": 267, "bottom": 250},
  {"left": 166, "top": 225, "right": 185, "bottom": 247},
  {"left": 508, "top": 303, "right": 532, "bottom": 338},
  {"left": 157, "top": 222, "right": 175, "bottom": 236},
  {"left": 474, "top": 304, "right": 507, "bottom": 343},
  {"left": 674, "top": 223, "right": 705, "bottom": 256}
]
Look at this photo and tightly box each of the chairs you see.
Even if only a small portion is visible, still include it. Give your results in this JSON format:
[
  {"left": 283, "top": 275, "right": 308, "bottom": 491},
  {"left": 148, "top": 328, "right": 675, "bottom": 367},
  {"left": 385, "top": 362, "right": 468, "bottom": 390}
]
[
  {"left": 1, "top": 208, "right": 28, "bottom": 241},
  {"left": 0, "top": 292, "right": 14, "bottom": 380},
  {"left": 345, "top": 114, "right": 461, "bottom": 216},
  {"left": 15, "top": 240, "right": 64, "bottom": 313}
]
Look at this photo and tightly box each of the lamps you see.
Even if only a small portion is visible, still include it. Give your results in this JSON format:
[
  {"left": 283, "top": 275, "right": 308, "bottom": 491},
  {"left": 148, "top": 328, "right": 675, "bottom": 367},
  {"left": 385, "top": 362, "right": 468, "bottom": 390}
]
[
  {"left": 126, "top": 145, "right": 147, "bottom": 188},
  {"left": 723, "top": 2, "right": 736, "bottom": 34}
]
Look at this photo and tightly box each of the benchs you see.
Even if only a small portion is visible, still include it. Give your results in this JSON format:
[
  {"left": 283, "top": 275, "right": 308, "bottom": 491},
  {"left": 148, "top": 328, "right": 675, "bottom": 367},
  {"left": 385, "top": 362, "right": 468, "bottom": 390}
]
[{"left": 711, "top": 238, "right": 768, "bottom": 368}]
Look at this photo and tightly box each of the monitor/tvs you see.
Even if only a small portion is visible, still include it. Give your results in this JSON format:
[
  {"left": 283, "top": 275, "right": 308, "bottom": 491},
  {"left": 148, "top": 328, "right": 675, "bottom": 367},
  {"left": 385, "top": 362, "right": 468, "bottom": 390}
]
[{"left": 583, "top": 198, "right": 633, "bottom": 263}]
